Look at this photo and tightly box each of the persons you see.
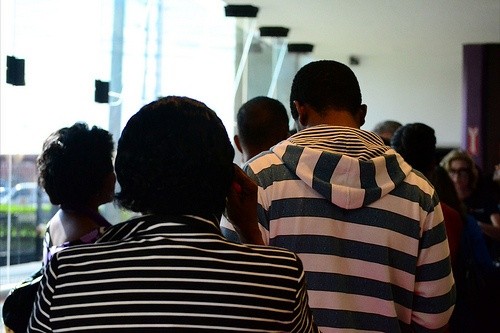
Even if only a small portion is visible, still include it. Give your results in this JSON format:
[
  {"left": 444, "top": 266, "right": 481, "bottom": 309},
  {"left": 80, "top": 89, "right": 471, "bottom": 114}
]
[
  {"left": 25, "top": 96, "right": 324, "bottom": 333},
  {"left": 220, "top": 60, "right": 456, "bottom": 333},
  {"left": 234, "top": 96, "right": 292, "bottom": 163},
  {"left": 370, "top": 121, "right": 500, "bottom": 333},
  {"left": 36, "top": 122, "right": 119, "bottom": 274}
]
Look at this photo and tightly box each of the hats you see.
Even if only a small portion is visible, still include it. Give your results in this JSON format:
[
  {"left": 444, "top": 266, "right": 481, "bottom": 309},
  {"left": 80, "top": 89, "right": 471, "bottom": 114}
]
[{"left": 391, "top": 123, "right": 436, "bottom": 154}]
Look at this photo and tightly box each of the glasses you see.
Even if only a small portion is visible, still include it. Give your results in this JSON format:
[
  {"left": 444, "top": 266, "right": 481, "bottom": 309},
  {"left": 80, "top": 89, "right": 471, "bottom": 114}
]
[{"left": 449, "top": 167, "right": 468, "bottom": 173}]
